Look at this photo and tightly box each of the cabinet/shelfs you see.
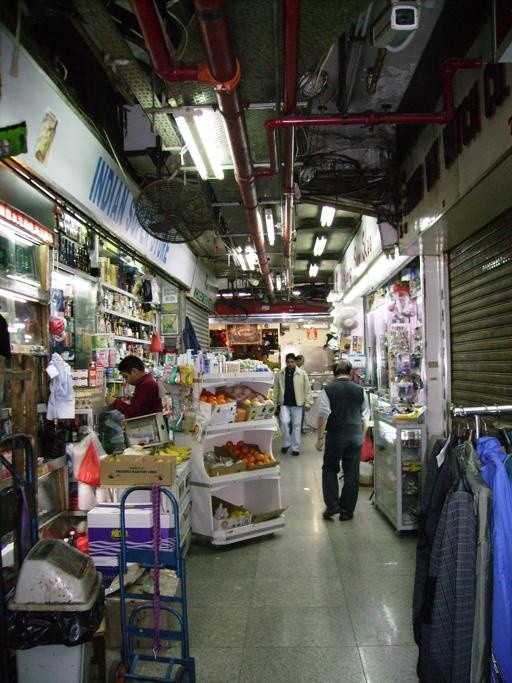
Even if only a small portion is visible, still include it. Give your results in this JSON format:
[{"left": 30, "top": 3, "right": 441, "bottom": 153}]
[
  {"left": 375, "top": 416, "right": 426, "bottom": 534},
  {"left": 100, "top": 279, "right": 159, "bottom": 384},
  {"left": 166, "top": 371, "right": 285, "bottom": 546}
]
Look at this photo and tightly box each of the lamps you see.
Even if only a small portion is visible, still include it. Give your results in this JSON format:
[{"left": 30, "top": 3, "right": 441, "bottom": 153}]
[
  {"left": 307, "top": 259, "right": 320, "bottom": 281},
  {"left": 313, "top": 231, "right": 328, "bottom": 257},
  {"left": 263, "top": 204, "right": 275, "bottom": 246},
  {"left": 174, "top": 110, "right": 224, "bottom": 180}
]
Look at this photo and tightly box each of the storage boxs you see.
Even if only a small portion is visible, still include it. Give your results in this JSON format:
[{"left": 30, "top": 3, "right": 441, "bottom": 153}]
[
  {"left": 105, "top": 566, "right": 181, "bottom": 650},
  {"left": 121, "top": 412, "right": 171, "bottom": 450},
  {"left": 100, "top": 455, "right": 176, "bottom": 486},
  {"left": 88, "top": 502, "right": 189, "bottom": 566}
]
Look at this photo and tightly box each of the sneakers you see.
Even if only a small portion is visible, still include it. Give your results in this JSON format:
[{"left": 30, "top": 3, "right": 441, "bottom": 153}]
[
  {"left": 339, "top": 512, "right": 353, "bottom": 521},
  {"left": 323, "top": 504, "right": 343, "bottom": 517},
  {"left": 281, "top": 447, "right": 288, "bottom": 453},
  {"left": 292, "top": 451, "right": 299, "bottom": 456}
]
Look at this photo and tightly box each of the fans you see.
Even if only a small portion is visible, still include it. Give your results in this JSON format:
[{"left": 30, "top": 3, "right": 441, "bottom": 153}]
[
  {"left": 134, "top": 179, "right": 214, "bottom": 243},
  {"left": 214, "top": 272, "right": 248, "bottom": 324}
]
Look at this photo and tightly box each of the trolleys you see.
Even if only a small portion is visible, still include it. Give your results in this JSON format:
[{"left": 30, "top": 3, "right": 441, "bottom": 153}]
[{"left": 108, "top": 483, "right": 198, "bottom": 683}]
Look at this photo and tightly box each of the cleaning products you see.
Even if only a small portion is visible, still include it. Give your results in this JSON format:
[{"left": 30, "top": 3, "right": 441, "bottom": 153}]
[{"left": 185, "top": 348, "right": 228, "bottom": 376}]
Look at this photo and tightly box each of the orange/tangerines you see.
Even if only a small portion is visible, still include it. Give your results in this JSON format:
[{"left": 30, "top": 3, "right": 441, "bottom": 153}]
[
  {"left": 222, "top": 440, "right": 271, "bottom": 467},
  {"left": 199, "top": 389, "right": 233, "bottom": 405}
]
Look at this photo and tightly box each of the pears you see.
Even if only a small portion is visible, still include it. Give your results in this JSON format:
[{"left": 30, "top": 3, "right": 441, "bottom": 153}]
[{"left": 222, "top": 510, "right": 250, "bottom": 520}]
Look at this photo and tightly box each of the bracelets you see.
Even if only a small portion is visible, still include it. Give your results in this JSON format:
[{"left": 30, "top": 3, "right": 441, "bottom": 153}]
[{"left": 316, "top": 436, "right": 324, "bottom": 440}]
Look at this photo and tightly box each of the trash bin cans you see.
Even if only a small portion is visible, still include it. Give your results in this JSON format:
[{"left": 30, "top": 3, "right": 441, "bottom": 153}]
[{"left": 8, "top": 538, "right": 105, "bottom": 683}]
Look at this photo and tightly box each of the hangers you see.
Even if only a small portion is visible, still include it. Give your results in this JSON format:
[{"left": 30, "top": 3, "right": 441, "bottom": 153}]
[{"left": 442, "top": 403, "right": 512, "bottom": 455}]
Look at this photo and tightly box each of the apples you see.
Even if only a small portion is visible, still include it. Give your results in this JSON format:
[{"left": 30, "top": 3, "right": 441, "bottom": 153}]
[{"left": 225, "top": 386, "right": 265, "bottom": 419}]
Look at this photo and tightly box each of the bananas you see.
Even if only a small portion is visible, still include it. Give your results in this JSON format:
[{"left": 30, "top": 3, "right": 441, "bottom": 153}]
[
  {"left": 104, "top": 454, "right": 120, "bottom": 464},
  {"left": 153, "top": 442, "right": 191, "bottom": 464}
]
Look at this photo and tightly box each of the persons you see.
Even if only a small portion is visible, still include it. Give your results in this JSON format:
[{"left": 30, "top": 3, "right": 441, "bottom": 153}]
[
  {"left": 261, "top": 353, "right": 313, "bottom": 455},
  {"left": 104, "top": 354, "right": 163, "bottom": 448},
  {"left": 313, "top": 358, "right": 371, "bottom": 522}
]
